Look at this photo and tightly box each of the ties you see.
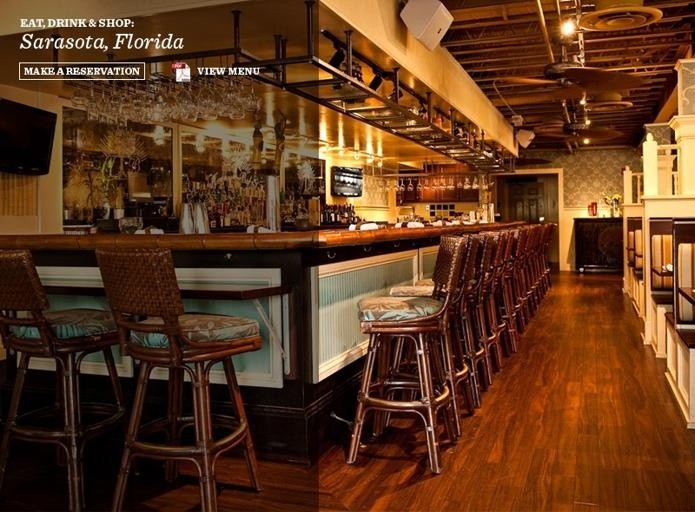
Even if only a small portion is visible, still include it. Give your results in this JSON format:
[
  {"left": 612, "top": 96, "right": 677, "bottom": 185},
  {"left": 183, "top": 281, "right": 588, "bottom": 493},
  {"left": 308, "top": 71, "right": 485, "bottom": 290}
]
[{"left": 0, "top": 97, "right": 59, "bottom": 177}]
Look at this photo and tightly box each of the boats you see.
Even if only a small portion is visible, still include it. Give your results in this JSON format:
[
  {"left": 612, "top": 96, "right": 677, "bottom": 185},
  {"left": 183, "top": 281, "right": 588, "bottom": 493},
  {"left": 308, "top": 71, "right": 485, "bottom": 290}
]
[
  {"left": 400, "top": 1, "right": 454, "bottom": 51},
  {"left": 515, "top": 129, "right": 535, "bottom": 149}
]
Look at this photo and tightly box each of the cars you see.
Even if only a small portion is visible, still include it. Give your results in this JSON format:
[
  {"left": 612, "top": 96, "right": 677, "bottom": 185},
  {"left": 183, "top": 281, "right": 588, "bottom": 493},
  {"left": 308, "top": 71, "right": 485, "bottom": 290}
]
[
  {"left": 375, "top": 179, "right": 382, "bottom": 192},
  {"left": 70, "top": 75, "right": 261, "bottom": 123},
  {"left": 457, "top": 177, "right": 463, "bottom": 188},
  {"left": 424, "top": 177, "right": 429, "bottom": 191},
  {"left": 384, "top": 178, "right": 391, "bottom": 192},
  {"left": 399, "top": 177, "right": 406, "bottom": 191},
  {"left": 406, "top": 178, "right": 414, "bottom": 192},
  {"left": 446, "top": 176, "right": 455, "bottom": 191},
  {"left": 463, "top": 176, "right": 472, "bottom": 189},
  {"left": 472, "top": 176, "right": 480, "bottom": 188},
  {"left": 438, "top": 176, "right": 445, "bottom": 191},
  {"left": 417, "top": 177, "right": 422, "bottom": 192},
  {"left": 391, "top": 177, "right": 399, "bottom": 191},
  {"left": 431, "top": 177, "right": 436, "bottom": 191},
  {"left": 480, "top": 174, "right": 488, "bottom": 191}
]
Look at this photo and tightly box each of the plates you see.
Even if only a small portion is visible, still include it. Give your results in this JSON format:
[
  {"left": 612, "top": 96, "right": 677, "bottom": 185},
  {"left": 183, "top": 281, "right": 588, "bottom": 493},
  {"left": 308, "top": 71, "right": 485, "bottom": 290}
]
[{"left": 573, "top": 217, "right": 623, "bottom": 275}]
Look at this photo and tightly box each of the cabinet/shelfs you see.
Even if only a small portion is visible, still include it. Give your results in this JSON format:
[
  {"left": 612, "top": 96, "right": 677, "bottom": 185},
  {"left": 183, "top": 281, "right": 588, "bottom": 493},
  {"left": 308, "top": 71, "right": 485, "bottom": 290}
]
[
  {"left": 342, "top": 61, "right": 362, "bottom": 81},
  {"left": 320, "top": 203, "right": 354, "bottom": 225},
  {"left": 62, "top": 191, "right": 69, "bottom": 225},
  {"left": 210, "top": 207, "right": 217, "bottom": 229}
]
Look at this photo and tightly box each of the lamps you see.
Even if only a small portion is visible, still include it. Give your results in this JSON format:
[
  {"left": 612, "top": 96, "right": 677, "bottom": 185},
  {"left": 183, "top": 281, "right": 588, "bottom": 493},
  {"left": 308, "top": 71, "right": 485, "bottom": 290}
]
[
  {"left": 633, "top": 217, "right": 642, "bottom": 318},
  {"left": 648, "top": 217, "right": 672, "bottom": 359},
  {"left": 385, "top": 233, "right": 476, "bottom": 437},
  {"left": 471, "top": 221, "right": 560, "bottom": 409},
  {"left": 95, "top": 247, "right": 265, "bottom": 511},
  {"left": 0, "top": 249, "right": 141, "bottom": 512},
  {"left": 672, "top": 217, "right": 695, "bottom": 431},
  {"left": 346, "top": 233, "right": 465, "bottom": 475},
  {"left": 627, "top": 217, "right": 634, "bottom": 298}
]
